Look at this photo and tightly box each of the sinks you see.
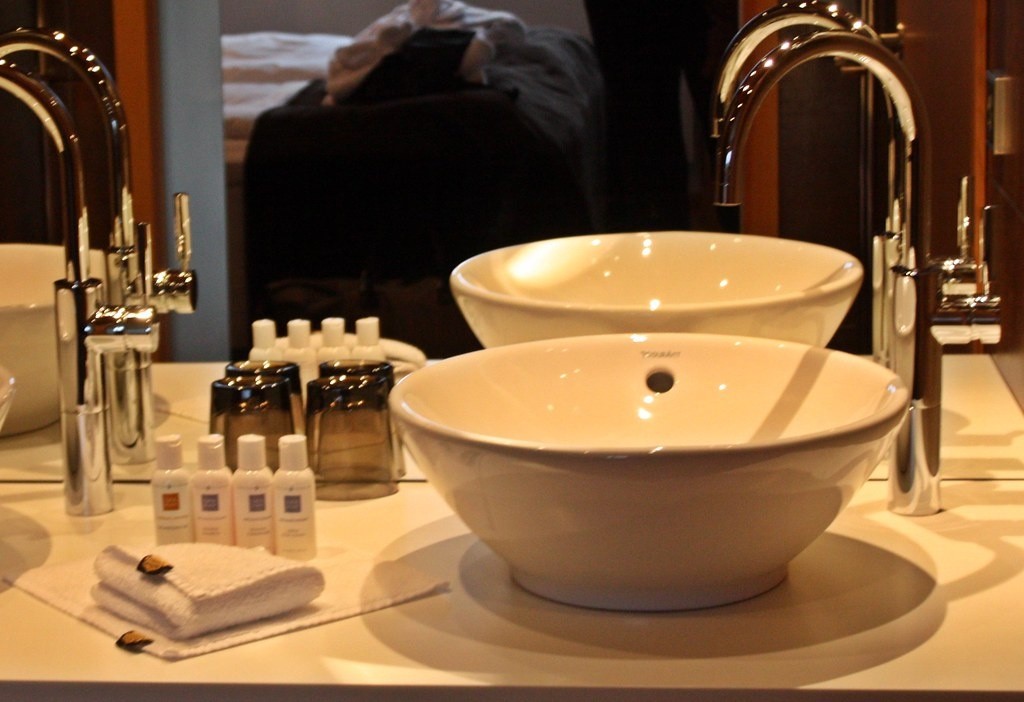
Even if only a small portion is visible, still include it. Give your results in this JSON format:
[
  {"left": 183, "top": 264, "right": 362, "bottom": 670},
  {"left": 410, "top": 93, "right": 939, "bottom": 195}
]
[
  {"left": 450, "top": 234, "right": 867, "bottom": 348},
  {"left": 1, "top": 241, "right": 126, "bottom": 441},
  {"left": 391, "top": 334, "right": 912, "bottom": 609}
]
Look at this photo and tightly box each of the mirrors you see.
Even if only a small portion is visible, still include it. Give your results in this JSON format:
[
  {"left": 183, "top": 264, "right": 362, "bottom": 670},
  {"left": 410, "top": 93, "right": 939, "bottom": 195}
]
[{"left": 0, "top": 0, "right": 1024, "bottom": 481}]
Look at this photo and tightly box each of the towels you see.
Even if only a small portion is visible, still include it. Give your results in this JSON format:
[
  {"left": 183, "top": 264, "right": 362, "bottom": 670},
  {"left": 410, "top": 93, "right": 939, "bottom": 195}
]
[{"left": 89, "top": 547, "right": 322, "bottom": 636}]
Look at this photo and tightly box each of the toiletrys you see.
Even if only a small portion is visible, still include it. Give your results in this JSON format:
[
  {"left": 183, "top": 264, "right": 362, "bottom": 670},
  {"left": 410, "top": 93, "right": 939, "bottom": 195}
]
[
  {"left": 158, "top": 437, "right": 322, "bottom": 554},
  {"left": 252, "top": 313, "right": 387, "bottom": 359}
]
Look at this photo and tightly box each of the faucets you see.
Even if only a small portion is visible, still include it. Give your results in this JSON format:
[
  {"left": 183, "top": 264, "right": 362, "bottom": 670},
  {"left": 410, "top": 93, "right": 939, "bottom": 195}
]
[
  {"left": 711, "top": 27, "right": 1003, "bottom": 520},
  {"left": 0, "top": 22, "right": 198, "bottom": 468},
  {"left": 712, "top": 1, "right": 999, "bottom": 375},
  {"left": 0, "top": 56, "right": 161, "bottom": 516}
]
